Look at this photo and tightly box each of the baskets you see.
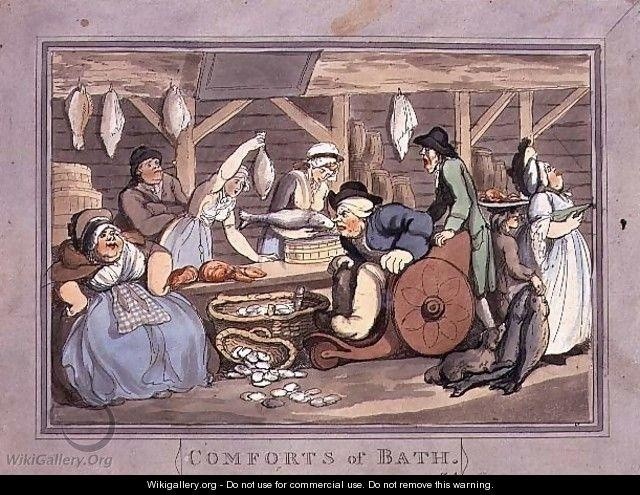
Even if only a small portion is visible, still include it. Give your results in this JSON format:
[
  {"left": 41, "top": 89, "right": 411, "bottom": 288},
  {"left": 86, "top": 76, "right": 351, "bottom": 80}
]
[
  {"left": 215, "top": 327, "right": 298, "bottom": 370},
  {"left": 205, "top": 289, "right": 331, "bottom": 351}
]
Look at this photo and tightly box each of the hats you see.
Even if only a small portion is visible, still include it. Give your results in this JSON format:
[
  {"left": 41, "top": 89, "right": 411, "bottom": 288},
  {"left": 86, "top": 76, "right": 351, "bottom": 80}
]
[
  {"left": 130, "top": 146, "right": 162, "bottom": 177},
  {"left": 328, "top": 181, "right": 384, "bottom": 211},
  {"left": 414, "top": 127, "right": 459, "bottom": 158}
]
